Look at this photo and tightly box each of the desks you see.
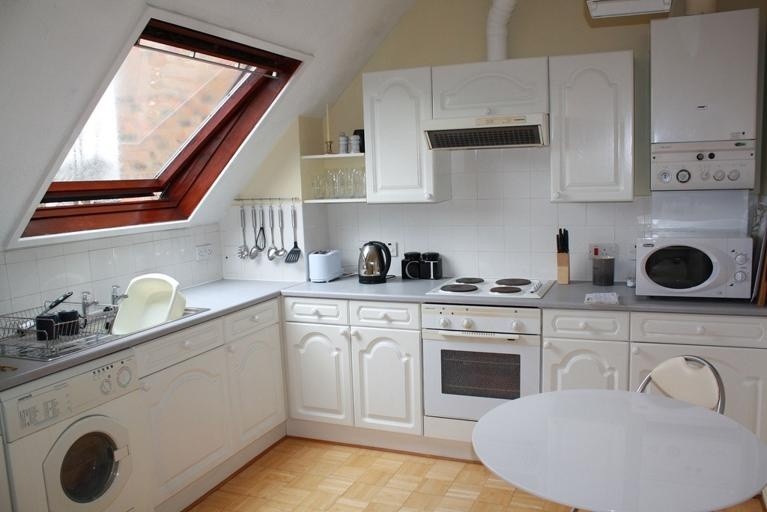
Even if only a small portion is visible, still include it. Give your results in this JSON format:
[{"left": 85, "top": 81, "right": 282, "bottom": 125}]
[{"left": 471, "top": 389, "right": 766, "bottom": 511}]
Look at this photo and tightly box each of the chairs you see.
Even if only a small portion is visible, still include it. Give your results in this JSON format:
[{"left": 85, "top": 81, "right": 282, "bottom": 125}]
[{"left": 636, "top": 355, "right": 725, "bottom": 415}]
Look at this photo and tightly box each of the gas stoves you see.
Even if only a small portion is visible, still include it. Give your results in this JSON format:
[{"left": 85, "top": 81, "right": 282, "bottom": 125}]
[{"left": 422, "top": 273, "right": 557, "bottom": 299}]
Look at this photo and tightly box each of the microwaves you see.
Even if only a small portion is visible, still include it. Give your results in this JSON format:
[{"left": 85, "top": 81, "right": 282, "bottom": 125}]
[{"left": 631, "top": 232, "right": 754, "bottom": 302}]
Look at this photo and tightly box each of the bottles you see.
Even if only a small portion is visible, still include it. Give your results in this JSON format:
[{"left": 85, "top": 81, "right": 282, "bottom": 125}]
[
  {"left": 309, "top": 166, "right": 366, "bottom": 200},
  {"left": 350, "top": 135, "right": 361, "bottom": 153},
  {"left": 401, "top": 252, "right": 422, "bottom": 280},
  {"left": 337, "top": 136, "right": 348, "bottom": 154},
  {"left": 419, "top": 252, "right": 443, "bottom": 281}
]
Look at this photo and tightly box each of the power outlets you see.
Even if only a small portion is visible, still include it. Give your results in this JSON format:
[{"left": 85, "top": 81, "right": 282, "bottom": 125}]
[
  {"left": 382, "top": 242, "right": 398, "bottom": 256},
  {"left": 195, "top": 245, "right": 213, "bottom": 261}
]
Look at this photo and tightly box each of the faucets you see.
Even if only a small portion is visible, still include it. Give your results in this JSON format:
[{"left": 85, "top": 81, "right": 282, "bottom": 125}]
[
  {"left": 111, "top": 285, "right": 128, "bottom": 313},
  {"left": 81, "top": 290, "right": 99, "bottom": 315}
]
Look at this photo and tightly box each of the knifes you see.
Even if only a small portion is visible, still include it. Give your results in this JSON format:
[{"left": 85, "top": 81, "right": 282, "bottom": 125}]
[
  {"left": 555, "top": 227, "right": 569, "bottom": 253},
  {"left": 36, "top": 291, "right": 73, "bottom": 316}
]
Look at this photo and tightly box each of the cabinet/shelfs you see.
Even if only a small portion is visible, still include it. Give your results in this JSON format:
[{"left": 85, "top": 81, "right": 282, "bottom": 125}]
[
  {"left": 541, "top": 308, "right": 629, "bottom": 389},
  {"left": 629, "top": 311, "right": 766, "bottom": 443},
  {"left": 131, "top": 318, "right": 240, "bottom": 512},
  {"left": 361, "top": 66, "right": 450, "bottom": 205},
  {"left": 281, "top": 296, "right": 423, "bottom": 455},
  {"left": 431, "top": 57, "right": 550, "bottom": 119},
  {"left": 299, "top": 152, "right": 367, "bottom": 205},
  {"left": 549, "top": 49, "right": 635, "bottom": 203},
  {"left": 222, "top": 296, "right": 286, "bottom": 472}
]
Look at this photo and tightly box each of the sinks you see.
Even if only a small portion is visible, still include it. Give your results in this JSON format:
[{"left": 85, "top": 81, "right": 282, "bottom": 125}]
[{"left": 81, "top": 307, "right": 209, "bottom": 336}]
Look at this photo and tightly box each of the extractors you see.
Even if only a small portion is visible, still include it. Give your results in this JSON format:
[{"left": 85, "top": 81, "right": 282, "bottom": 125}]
[{"left": 424, "top": 112, "right": 548, "bottom": 153}]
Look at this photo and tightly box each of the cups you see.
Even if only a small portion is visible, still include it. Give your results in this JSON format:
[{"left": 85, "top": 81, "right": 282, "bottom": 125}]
[
  {"left": 592, "top": 257, "right": 614, "bottom": 287},
  {"left": 34, "top": 310, "right": 87, "bottom": 341}
]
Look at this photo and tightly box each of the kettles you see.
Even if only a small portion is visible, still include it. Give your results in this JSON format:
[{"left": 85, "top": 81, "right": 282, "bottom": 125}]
[{"left": 356, "top": 240, "right": 392, "bottom": 284}]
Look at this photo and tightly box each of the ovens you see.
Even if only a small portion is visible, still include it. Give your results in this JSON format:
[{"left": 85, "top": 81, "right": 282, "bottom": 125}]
[{"left": 418, "top": 302, "right": 543, "bottom": 422}]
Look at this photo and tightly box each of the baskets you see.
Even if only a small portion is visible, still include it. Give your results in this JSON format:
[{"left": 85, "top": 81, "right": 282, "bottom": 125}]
[{"left": 0, "top": 300, "right": 120, "bottom": 362}]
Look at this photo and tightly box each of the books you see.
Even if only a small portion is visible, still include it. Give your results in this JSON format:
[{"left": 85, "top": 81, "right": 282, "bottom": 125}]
[{"left": 584, "top": 292, "right": 619, "bottom": 304}]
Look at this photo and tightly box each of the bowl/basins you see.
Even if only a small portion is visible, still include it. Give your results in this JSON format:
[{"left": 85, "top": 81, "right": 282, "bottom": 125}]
[{"left": 108, "top": 271, "right": 187, "bottom": 338}]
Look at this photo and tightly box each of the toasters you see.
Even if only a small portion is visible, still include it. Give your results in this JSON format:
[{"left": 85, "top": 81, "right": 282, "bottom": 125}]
[{"left": 308, "top": 246, "right": 343, "bottom": 284}]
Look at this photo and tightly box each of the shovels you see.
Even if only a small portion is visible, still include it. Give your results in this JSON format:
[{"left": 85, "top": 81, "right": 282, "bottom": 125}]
[{"left": 285, "top": 209, "right": 301, "bottom": 263}]
